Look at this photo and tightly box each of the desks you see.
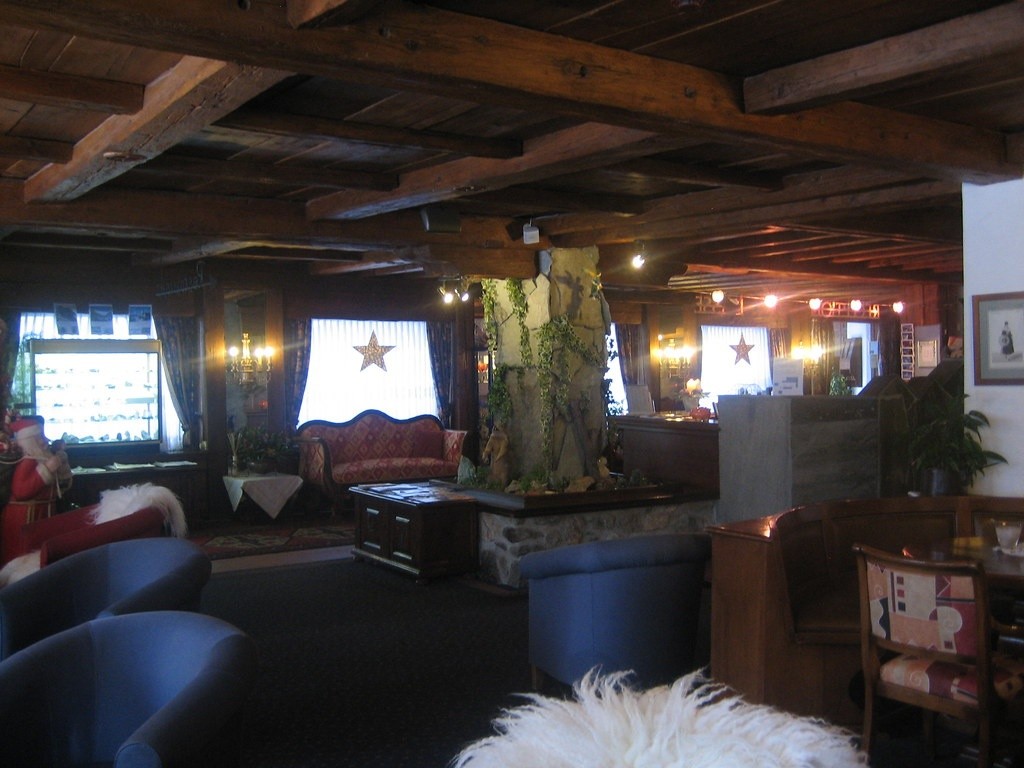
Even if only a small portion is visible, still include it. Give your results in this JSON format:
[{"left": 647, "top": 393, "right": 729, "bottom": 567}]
[
  {"left": 901, "top": 544, "right": 1024, "bottom": 637},
  {"left": 221, "top": 476, "right": 306, "bottom": 524},
  {"left": 69, "top": 451, "right": 198, "bottom": 530},
  {"left": 347, "top": 481, "right": 479, "bottom": 584}
]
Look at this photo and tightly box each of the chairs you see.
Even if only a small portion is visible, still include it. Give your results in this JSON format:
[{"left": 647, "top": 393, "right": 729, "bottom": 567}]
[
  {"left": 18, "top": 503, "right": 165, "bottom": 570},
  {"left": 0, "top": 607, "right": 264, "bottom": 768},
  {"left": 0, "top": 535, "right": 212, "bottom": 663},
  {"left": 849, "top": 545, "right": 1024, "bottom": 767},
  {"left": 518, "top": 531, "right": 710, "bottom": 701}
]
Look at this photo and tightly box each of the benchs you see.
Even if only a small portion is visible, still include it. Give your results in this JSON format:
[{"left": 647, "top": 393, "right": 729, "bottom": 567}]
[{"left": 709, "top": 493, "right": 1024, "bottom": 731}]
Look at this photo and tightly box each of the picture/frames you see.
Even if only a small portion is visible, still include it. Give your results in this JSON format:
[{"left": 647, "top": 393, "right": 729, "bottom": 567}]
[{"left": 971, "top": 292, "right": 1024, "bottom": 385}]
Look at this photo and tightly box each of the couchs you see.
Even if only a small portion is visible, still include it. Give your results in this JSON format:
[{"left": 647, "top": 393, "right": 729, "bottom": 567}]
[{"left": 297, "top": 408, "right": 473, "bottom": 519}]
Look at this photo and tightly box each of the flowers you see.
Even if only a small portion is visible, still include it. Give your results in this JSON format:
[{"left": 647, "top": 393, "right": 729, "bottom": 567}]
[{"left": 677, "top": 378, "right": 711, "bottom": 399}]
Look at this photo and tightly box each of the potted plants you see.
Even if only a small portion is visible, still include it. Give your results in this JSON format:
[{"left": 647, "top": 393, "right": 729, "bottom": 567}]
[
  {"left": 902, "top": 387, "right": 1010, "bottom": 495},
  {"left": 225, "top": 426, "right": 300, "bottom": 478}
]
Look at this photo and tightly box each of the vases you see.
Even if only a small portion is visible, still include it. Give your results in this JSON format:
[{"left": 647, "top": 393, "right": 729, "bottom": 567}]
[{"left": 682, "top": 396, "right": 699, "bottom": 413}]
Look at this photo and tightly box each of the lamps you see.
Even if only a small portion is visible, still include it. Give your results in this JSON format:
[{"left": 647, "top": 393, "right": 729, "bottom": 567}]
[
  {"left": 654, "top": 347, "right": 692, "bottom": 380},
  {"left": 229, "top": 332, "right": 272, "bottom": 390},
  {"left": 632, "top": 240, "right": 645, "bottom": 268},
  {"left": 420, "top": 207, "right": 461, "bottom": 235}
]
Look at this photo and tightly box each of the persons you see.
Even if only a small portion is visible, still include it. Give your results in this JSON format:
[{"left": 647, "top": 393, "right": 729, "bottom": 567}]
[
  {"left": 3, "top": 416, "right": 74, "bottom": 529},
  {"left": 1001, "top": 321, "right": 1014, "bottom": 360}
]
